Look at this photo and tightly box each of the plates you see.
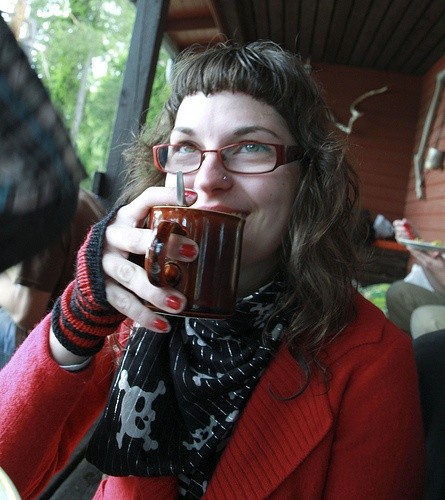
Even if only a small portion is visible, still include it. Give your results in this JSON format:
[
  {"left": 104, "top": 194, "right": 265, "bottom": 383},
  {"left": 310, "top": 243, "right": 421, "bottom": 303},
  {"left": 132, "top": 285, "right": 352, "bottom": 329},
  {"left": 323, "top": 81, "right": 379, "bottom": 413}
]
[{"left": 396, "top": 238, "right": 445, "bottom": 253}]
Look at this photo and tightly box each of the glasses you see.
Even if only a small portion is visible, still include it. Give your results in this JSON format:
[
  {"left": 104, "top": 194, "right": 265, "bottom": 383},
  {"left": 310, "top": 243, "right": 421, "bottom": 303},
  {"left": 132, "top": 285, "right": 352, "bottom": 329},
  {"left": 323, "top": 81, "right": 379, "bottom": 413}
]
[{"left": 152, "top": 144, "right": 316, "bottom": 174}]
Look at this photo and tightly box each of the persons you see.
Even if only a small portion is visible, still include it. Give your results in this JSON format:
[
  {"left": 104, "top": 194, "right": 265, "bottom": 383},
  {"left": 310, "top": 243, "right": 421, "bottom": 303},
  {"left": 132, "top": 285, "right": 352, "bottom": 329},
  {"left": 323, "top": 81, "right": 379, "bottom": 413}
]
[
  {"left": 0, "top": 37, "right": 417, "bottom": 500},
  {"left": 386, "top": 247, "right": 445, "bottom": 339}
]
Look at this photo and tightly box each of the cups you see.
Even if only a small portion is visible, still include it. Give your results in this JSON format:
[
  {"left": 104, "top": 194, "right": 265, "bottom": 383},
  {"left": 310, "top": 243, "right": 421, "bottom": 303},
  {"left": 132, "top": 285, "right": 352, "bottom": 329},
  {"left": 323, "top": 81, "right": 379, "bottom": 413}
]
[{"left": 142, "top": 205, "right": 246, "bottom": 321}]
